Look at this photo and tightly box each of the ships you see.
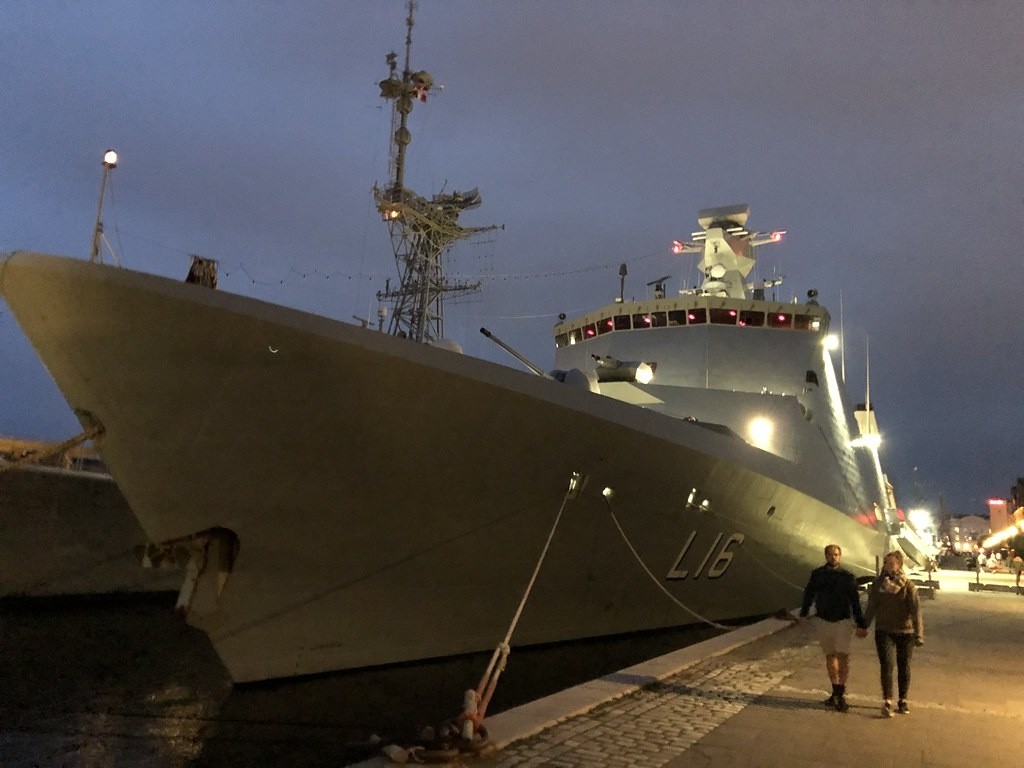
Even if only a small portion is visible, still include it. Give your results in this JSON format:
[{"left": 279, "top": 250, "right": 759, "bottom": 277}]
[{"left": 3, "top": 2, "right": 943, "bottom": 695}]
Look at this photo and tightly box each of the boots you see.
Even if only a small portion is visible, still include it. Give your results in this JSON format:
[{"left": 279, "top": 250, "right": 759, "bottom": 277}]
[
  {"left": 823, "top": 683, "right": 839, "bottom": 706},
  {"left": 834, "top": 685, "right": 849, "bottom": 712}
]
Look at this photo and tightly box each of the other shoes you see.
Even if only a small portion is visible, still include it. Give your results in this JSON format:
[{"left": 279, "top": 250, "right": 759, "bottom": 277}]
[
  {"left": 898, "top": 700, "right": 909, "bottom": 714},
  {"left": 879, "top": 703, "right": 894, "bottom": 717}
]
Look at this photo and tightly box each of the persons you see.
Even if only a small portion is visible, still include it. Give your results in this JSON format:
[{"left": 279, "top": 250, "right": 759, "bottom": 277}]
[{"left": 799, "top": 545, "right": 924, "bottom": 717}]
